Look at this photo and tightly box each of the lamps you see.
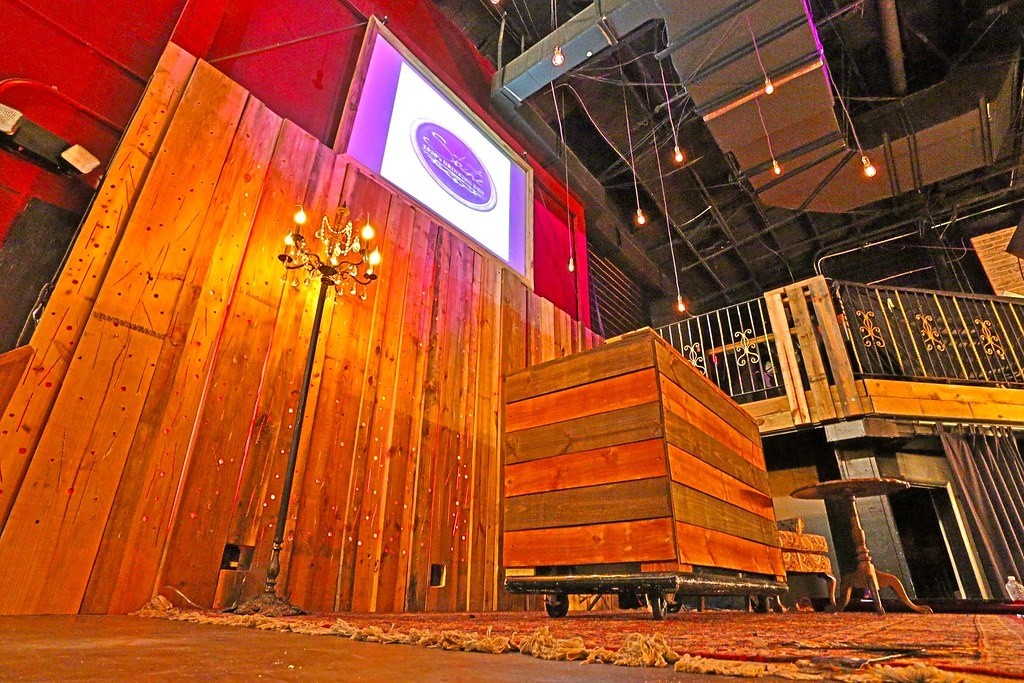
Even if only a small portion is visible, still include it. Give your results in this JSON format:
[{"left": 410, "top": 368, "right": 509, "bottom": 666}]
[{"left": 221, "top": 199, "right": 383, "bottom": 619}]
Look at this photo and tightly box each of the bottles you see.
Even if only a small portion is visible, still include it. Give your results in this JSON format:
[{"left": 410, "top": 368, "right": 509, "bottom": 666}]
[{"left": 1005, "top": 574, "right": 1024, "bottom": 618}]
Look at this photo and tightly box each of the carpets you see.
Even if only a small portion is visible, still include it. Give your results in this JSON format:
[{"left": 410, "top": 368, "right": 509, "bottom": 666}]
[{"left": 125, "top": 594, "right": 1024, "bottom": 683}]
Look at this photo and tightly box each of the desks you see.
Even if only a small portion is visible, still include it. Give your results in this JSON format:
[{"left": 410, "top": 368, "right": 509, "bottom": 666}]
[{"left": 787, "top": 477, "right": 933, "bottom": 617}]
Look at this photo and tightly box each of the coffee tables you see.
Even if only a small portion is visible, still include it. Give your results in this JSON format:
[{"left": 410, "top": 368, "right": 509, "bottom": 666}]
[{"left": 777, "top": 529, "right": 839, "bottom": 614}]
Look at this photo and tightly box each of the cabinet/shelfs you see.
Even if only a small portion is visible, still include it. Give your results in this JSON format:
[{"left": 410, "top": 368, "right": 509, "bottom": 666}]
[{"left": 497, "top": 327, "right": 792, "bottom": 623}]
